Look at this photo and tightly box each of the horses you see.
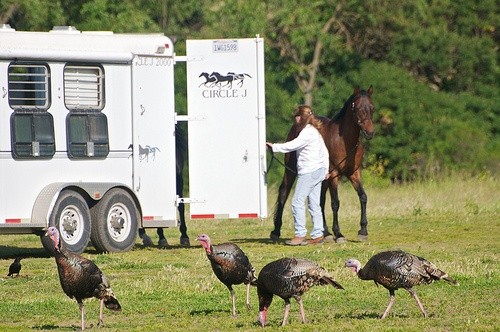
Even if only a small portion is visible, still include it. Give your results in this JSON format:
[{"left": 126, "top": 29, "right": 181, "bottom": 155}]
[
  {"left": 269, "top": 83, "right": 377, "bottom": 245},
  {"left": 136, "top": 114, "right": 192, "bottom": 248}
]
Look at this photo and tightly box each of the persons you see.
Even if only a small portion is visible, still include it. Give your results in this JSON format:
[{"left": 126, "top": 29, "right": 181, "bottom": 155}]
[{"left": 266, "top": 106, "right": 330, "bottom": 246}]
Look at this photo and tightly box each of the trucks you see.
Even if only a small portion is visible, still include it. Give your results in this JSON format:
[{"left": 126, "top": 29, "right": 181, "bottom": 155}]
[{"left": 0, "top": 23, "right": 267, "bottom": 256}]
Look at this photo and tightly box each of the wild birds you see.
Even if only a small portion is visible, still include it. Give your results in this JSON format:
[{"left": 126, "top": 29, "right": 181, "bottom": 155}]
[{"left": 6, "top": 255, "right": 26, "bottom": 277}]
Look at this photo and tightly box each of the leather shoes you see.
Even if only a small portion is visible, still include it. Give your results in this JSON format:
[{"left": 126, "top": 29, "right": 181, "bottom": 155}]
[
  {"left": 306, "top": 236, "right": 325, "bottom": 244},
  {"left": 285, "top": 236, "right": 306, "bottom": 246}
]
